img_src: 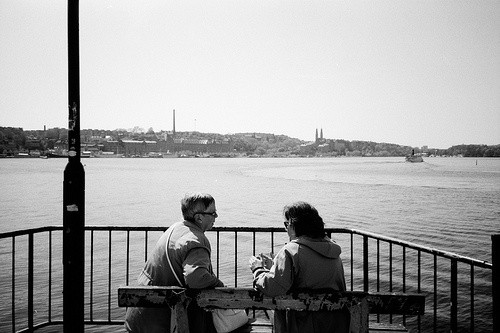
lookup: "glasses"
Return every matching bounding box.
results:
[284,221,290,228]
[193,210,216,218]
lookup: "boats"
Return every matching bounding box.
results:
[405,152,426,163]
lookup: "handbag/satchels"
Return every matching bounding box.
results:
[190,305,249,333]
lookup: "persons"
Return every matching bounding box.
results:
[247,199,351,333]
[123,192,251,333]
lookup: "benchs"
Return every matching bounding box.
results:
[117,286,427,333]
